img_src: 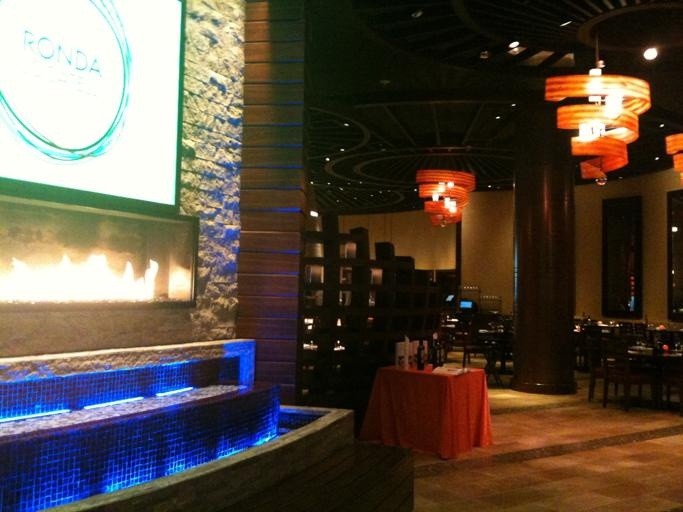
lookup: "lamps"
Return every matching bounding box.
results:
[413,150,476,230]
[542,32,653,187]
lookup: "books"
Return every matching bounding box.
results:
[431,363,470,376]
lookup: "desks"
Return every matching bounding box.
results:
[375,361,486,450]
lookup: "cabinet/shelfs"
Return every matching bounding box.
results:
[296,213,445,407]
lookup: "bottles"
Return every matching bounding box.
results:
[416,338,425,369]
[432,339,446,368]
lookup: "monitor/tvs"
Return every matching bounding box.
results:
[444,294,455,302]
[0,0,186,216]
[459,300,472,309]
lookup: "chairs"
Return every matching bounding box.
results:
[457,314,682,414]
